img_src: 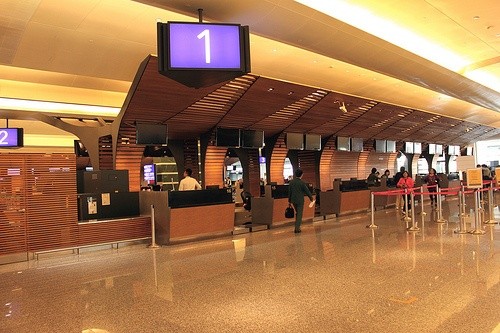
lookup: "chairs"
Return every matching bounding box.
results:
[241,191,255,212]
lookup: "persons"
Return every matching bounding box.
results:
[477,164,500,202]
[179,169,202,191]
[366,167,441,214]
[288,169,313,234]
[227,177,231,187]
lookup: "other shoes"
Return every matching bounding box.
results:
[402,211,405,215]
[435,204,437,206]
[408,209,412,213]
[431,201,433,204]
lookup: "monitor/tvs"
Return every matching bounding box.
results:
[74,140,89,157]
[336,136,351,151]
[157,21,251,88]
[135,124,168,146]
[241,130,264,148]
[386,141,396,153]
[0,128,23,148]
[143,164,156,185]
[305,133,321,151]
[350,138,363,152]
[405,142,422,154]
[215,127,241,148]
[373,140,386,153]
[428,144,474,156]
[285,133,304,150]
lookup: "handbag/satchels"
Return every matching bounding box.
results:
[285,205,294,218]
